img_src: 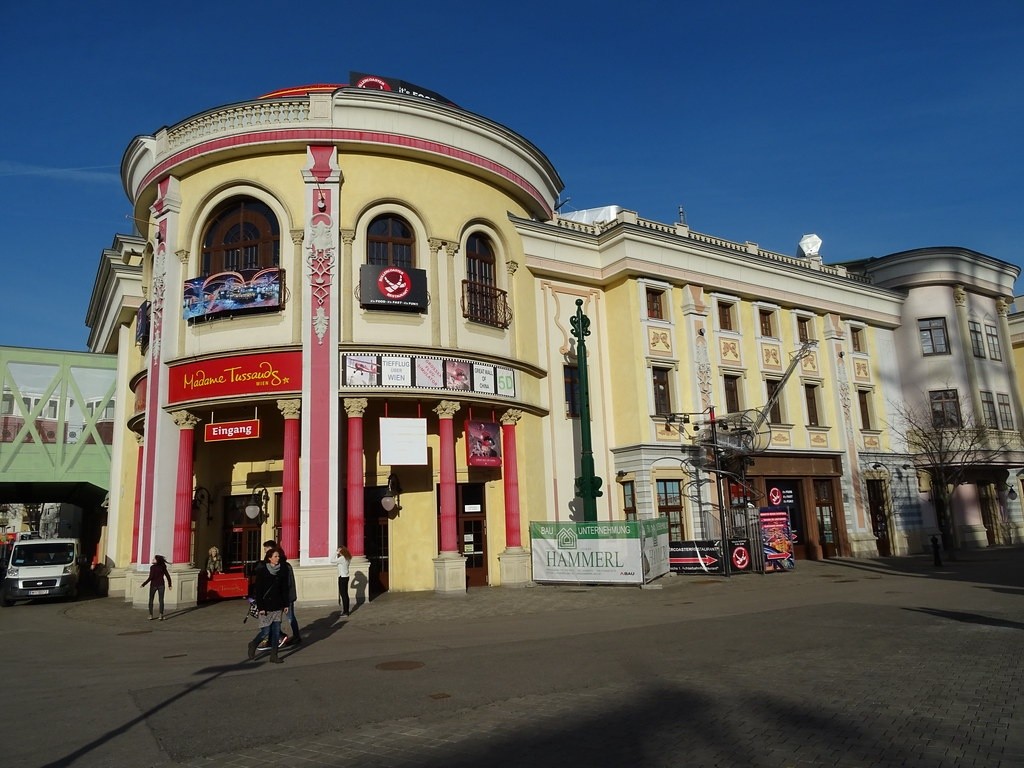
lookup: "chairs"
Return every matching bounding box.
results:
[52,548,69,559]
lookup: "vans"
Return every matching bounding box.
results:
[0,538,83,607]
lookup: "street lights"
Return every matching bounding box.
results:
[711,420,732,576]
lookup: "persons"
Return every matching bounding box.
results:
[140,555,172,620]
[205,547,225,579]
[930,534,944,567]
[248,548,290,663]
[256,540,302,650]
[330,545,352,617]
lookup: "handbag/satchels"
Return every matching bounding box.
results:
[250,603,259,618]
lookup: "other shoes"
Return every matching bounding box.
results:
[278,633,288,647]
[338,613,348,617]
[257,640,272,651]
[158,613,163,620]
[270,654,283,663]
[247,641,257,659]
[147,615,153,619]
[288,637,302,646]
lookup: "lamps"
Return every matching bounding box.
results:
[246,483,269,524]
[996,480,1017,500]
[901,464,910,470]
[872,464,881,470]
[381,473,402,517]
[192,486,214,526]
[618,470,627,477]
